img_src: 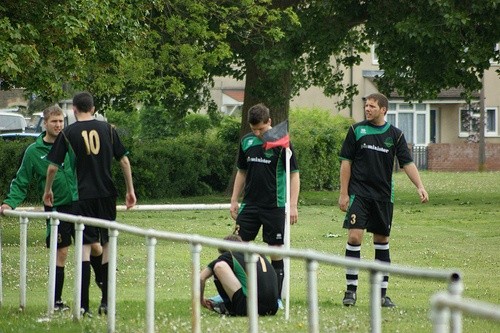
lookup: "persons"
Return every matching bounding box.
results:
[44,92,137,314]
[200,234,279,316]
[339,93,429,306]
[231,103,300,316]
[0,102,113,313]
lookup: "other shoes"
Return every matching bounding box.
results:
[208,296,224,305]
[53,301,70,312]
[343,290,357,305]
[381,296,395,306]
[213,302,230,316]
[278,299,284,309]
[81,308,93,319]
[99,304,107,315]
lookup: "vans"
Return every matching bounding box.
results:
[0,111,67,134]
